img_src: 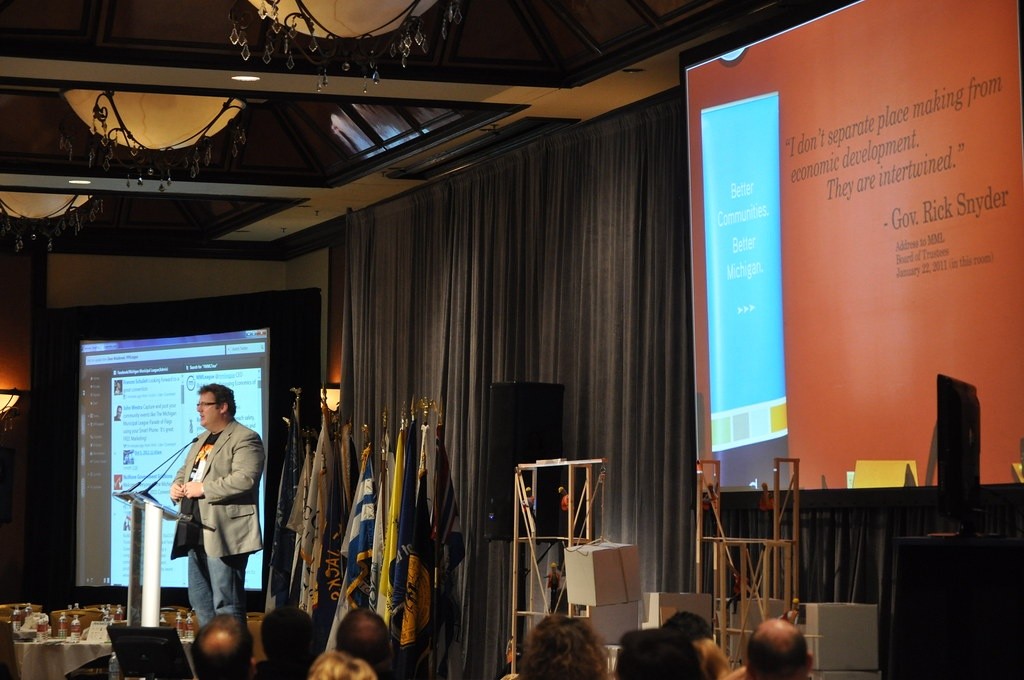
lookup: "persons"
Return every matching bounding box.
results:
[114,405,122,421]
[170,384,265,625]
[703,484,718,537]
[558,487,569,511]
[188,604,395,680]
[760,483,774,534]
[779,599,799,625]
[544,563,560,611]
[517,611,813,680]
[726,572,748,614]
[506,636,512,674]
[522,487,535,526]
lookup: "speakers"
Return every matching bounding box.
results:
[483,382,565,544]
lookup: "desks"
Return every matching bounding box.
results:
[888,536,1024,680]
[12,626,199,680]
[702,482,1024,623]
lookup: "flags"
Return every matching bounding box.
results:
[266,405,465,669]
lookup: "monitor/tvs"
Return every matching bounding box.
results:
[926,374,1003,537]
[107,624,194,680]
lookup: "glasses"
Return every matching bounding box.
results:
[197,402,222,407]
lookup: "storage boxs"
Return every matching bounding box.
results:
[564,542,883,680]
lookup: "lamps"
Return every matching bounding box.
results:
[0,190,105,251]
[57,87,248,191]
[228,0,466,94]
[0,387,25,431]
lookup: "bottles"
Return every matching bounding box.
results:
[109,652,120,680]
[25,603,32,618]
[185,613,194,640]
[58,613,68,641]
[13,606,21,632]
[68,605,72,610]
[116,605,123,614]
[73,603,80,610]
[71,615,81,643]
[106,604,111,611]
[101,605,105,611]
[102,611,110,622]
[160,615,166,622]
[176,612,183,639]
[37,614,48,642]
[246,613,249,621]
[110,615,114,622]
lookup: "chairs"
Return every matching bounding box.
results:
[0,603,268,680]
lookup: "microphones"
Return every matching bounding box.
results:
[121,437,199,505]
